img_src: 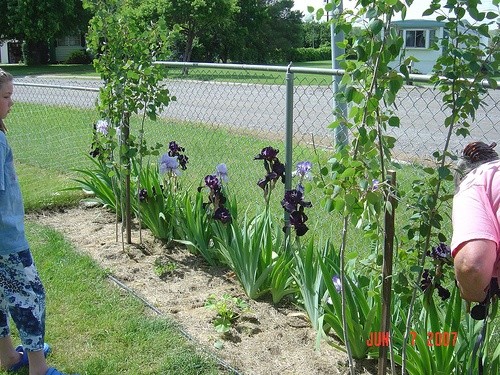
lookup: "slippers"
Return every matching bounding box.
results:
[6,343,51,372]
[46,365,79,375]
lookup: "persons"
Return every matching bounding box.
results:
[0,69,64,375]
[450,141,500,304]
